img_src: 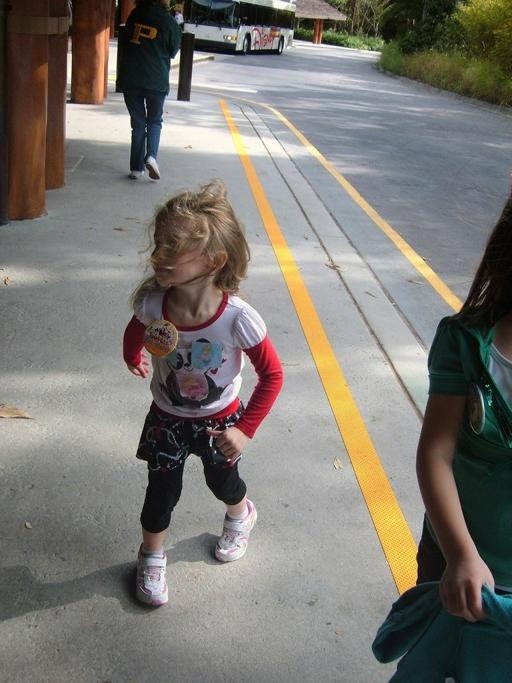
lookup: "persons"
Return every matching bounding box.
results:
[174,8,184,32]
[112,0,182,180]
[413,188,510,637]
[121,177,285,612]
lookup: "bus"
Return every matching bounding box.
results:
[185,0,297,56]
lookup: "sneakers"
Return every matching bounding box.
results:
[145,155,161,180]
[135,542,168,606]
[214,498,256,562]
[131,170,145,178]
